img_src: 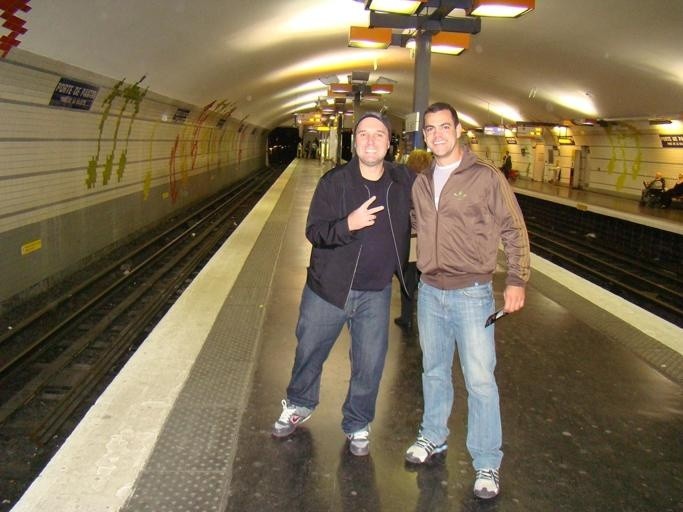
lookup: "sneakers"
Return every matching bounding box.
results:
[403,430,450,465]
[344,422,370,457]
[471,465,500,500]
[270,402,315,440]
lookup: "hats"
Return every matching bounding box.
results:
[353,111,393,144]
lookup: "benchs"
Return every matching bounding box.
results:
[641,190,683,210]
[331,157,348,166]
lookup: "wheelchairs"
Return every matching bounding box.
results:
[640,179,671,209]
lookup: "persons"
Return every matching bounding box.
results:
[309,142,318,157]
[304,140,310,158]
[404,102,532,499]
[395,149,431,327]
[271,111,418,459]
[297,140,303,158]
[501,150,512,178]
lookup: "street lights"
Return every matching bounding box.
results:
[348,0,535,153]
[322,81,393,159]
